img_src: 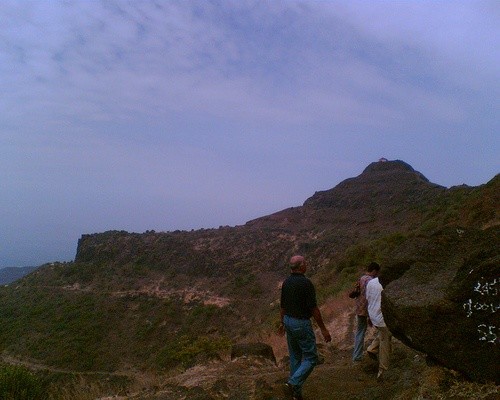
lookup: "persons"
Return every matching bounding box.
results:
[278,255,332,400]
[365,277,392,380]
[352,262,380,361]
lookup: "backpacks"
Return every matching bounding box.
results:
[348,276,368,299]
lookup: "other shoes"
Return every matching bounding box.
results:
[292,385,303,400]
[281,383,295,399]
